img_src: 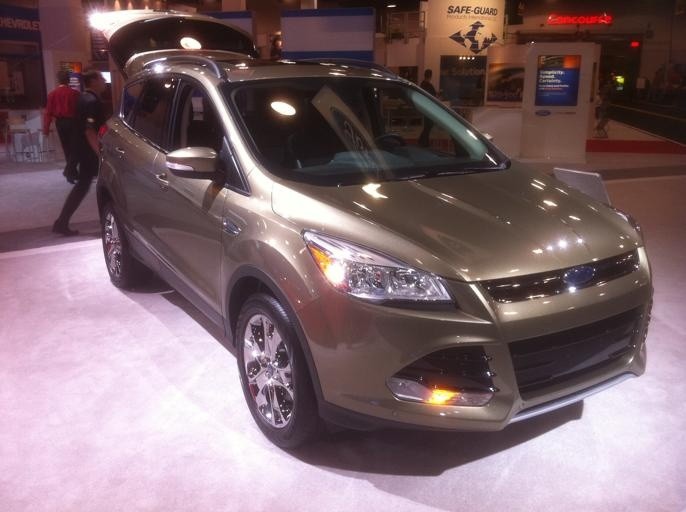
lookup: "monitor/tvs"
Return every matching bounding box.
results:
[440,55,486,100]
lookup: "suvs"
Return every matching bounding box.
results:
[88,4,657,454]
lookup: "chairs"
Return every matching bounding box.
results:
[0,110,57,164]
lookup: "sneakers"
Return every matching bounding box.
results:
[53,221,78,235]
[63,168,78,185]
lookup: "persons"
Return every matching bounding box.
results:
[51,69,107,239]
[415,69,438,149]
[39,71,82,185]
